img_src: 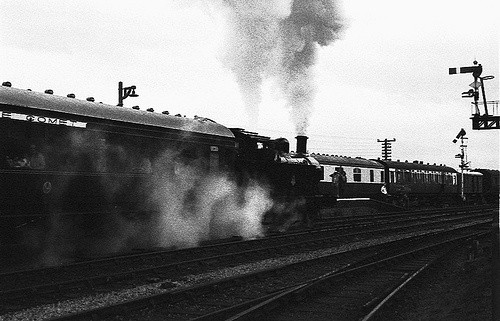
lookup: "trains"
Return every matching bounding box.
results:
[308,153,499,207]
[0,82,337,261]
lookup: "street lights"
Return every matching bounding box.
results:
[117,81,138,106]
